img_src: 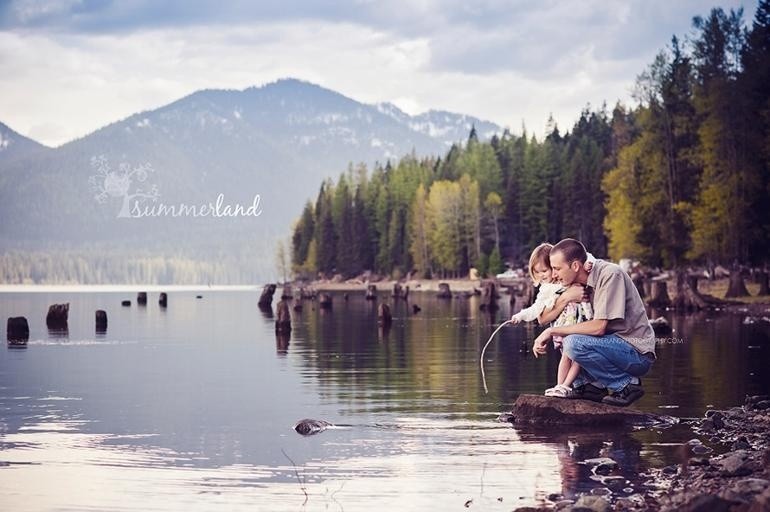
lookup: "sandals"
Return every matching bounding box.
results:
[545,383,573,398]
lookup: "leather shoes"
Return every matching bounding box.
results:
[568,383,644,406]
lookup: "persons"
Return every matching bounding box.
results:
[509,238,657,406]
